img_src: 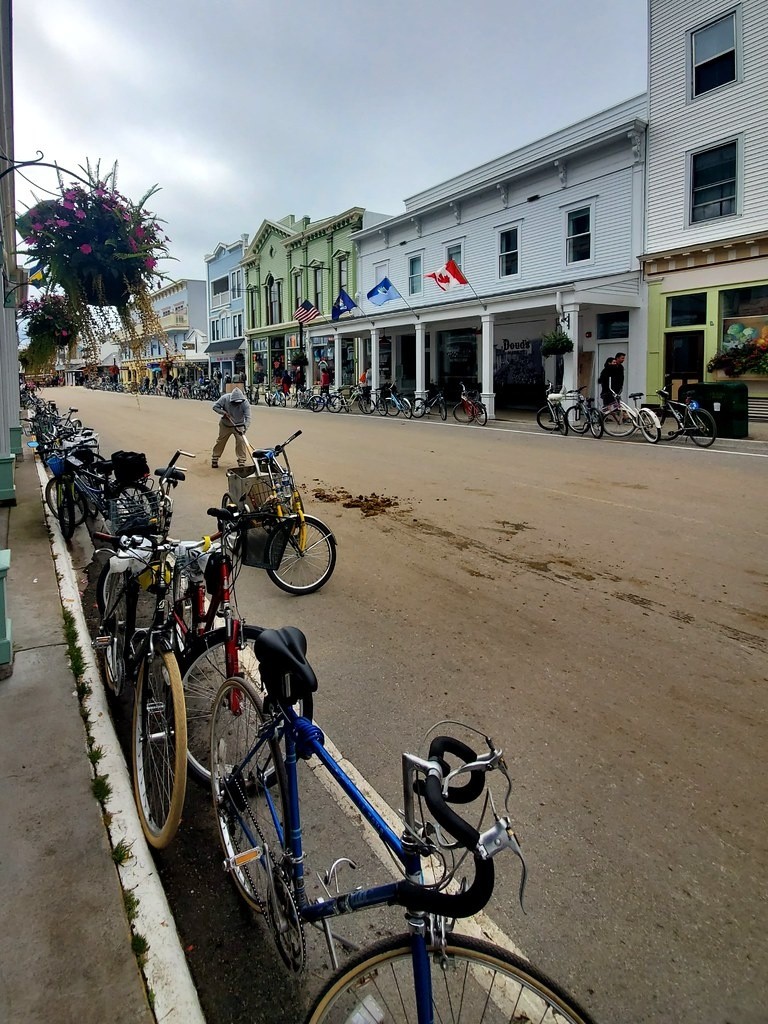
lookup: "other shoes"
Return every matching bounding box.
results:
[212,462,218,468]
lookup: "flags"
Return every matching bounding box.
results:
[424,259,467,292]
[30,261,48,289]
[367,276,401,305]
[331,288,358,321]
[292,299,320,323]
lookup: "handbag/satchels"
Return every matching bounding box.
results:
[111,450,150,483]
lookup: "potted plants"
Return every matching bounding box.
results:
[291,352,308,366]
[541,331,574,357]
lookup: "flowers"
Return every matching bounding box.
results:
[6,155,205,380]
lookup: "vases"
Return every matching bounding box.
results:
[20,358,28,366]
[54,329,71,347]
[82,276,129,308]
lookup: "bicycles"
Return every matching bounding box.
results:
[452,380,487,426]
[307,383,351,413]
[642,382,718,449]
[410,389,447,421]
[208,624,599,1024]
[217,429,337,595]
[564,385,605,439]
[19,374,323,853]
[324,382,368,413]
[536,380,569,436]
[357,387,388,417]
[383,378,412,419]
[600,377,662,444]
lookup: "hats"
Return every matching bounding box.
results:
[235,399,242,402]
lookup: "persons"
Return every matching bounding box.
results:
[19,374,64,392]
[86,366,247,394]
[359,368,371,405]
[212,387,251,469]
[254,359,330,403]
[600,352,632,425]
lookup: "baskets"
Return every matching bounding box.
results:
[22,423,36,436]
[109,489,161,534]
[351,387,363,396]
[47,456,69,483]
[248,476,286,510]
